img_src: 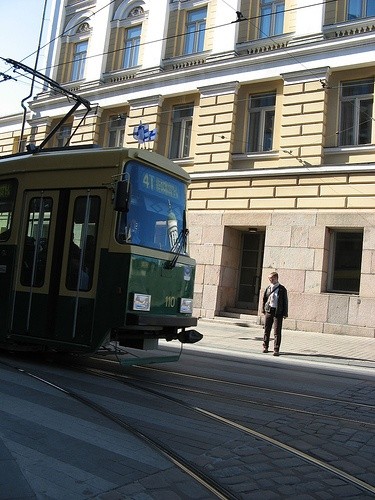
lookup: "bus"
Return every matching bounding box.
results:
[0,141,205,368]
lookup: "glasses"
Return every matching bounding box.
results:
[268,277,275,279]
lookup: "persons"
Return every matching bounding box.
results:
[261,271,288,357]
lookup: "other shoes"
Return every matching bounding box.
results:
[273,351,280,356]
[263,349,268,353]
